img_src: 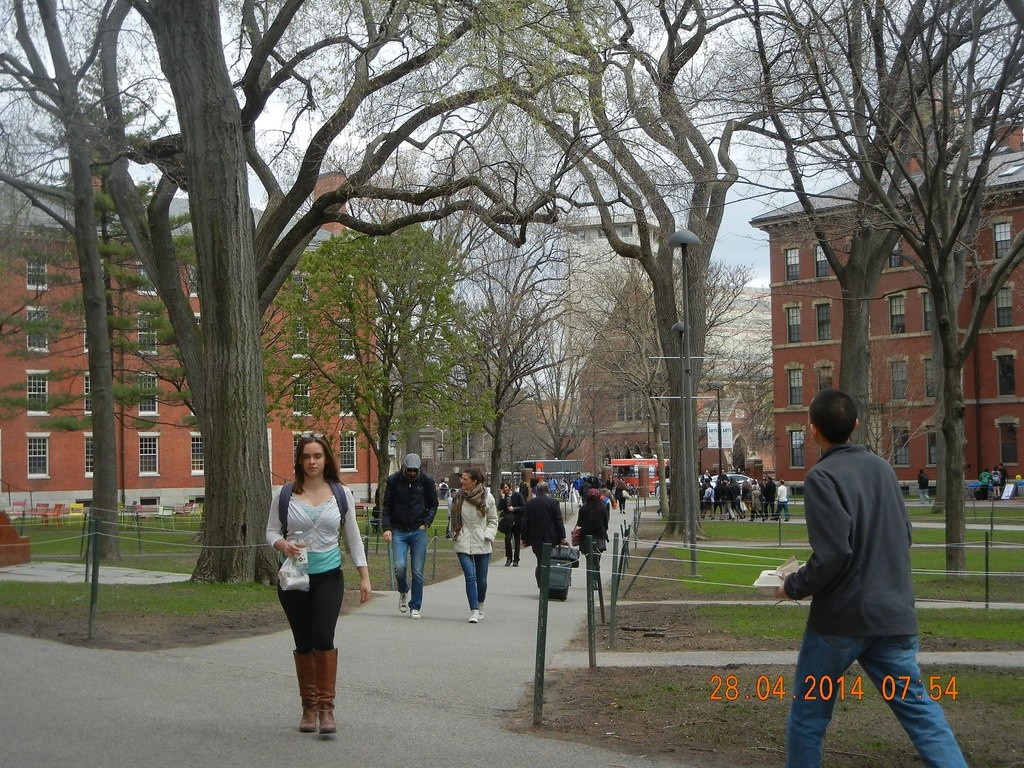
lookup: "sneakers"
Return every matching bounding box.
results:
[399,592,407,612]
[410,609,421,619]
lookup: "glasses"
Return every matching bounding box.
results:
[407,468,419,472]
[299,432,326,439]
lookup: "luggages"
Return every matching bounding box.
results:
[547,543,572,601]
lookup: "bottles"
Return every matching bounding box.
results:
[293,531,308,569]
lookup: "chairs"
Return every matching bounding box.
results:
[11,498,204,531]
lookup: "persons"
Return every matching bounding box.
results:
[656,486,661,516]
[530,473,539,495]
[918,469,931,504]
[577,489,609,590]
[497,482,529,566]
[446,489,456,540]
[265,436,371,734]
[979,463,1007,499]
[520,482,566,565]
[383,453,439,620]
[545,472,630,514]
[449,469,498,623]
[435,480,448,500]
[699,470,790,521]
[774,388,967,768]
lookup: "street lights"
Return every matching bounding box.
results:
[668,228,703,574]
[427,424,449,461]
[669,319,695,552]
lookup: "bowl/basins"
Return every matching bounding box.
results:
[753,570,784,595]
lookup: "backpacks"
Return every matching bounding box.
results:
[600,488,609,504]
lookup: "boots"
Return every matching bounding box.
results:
[313,648,338,732]
[293,649,318,732]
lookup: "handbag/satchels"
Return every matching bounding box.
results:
[552,541,580,568]
[613,500,618,510]
[740,500,747,512]
[497,515,515,533]
[622,490,630,498]
[702,497,711,506]
[571,524,582,546]
[278,556,310,592]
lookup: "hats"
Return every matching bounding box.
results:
[404,453,422,468]
[587,488,598,498]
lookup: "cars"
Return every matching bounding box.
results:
[699,473,759,490]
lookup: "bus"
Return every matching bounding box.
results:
[611,458,670,495]
[515,460,584,486]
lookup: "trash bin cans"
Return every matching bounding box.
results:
[927,486,937,497]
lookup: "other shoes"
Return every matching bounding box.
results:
[699,513,790,522]
[469,610,479,623]
[620,511,622,513]
[505,557,513,566]
[593,581,598,591]
[513,559,519,567]
[623,511,626,514]
[478,602,485,619]
